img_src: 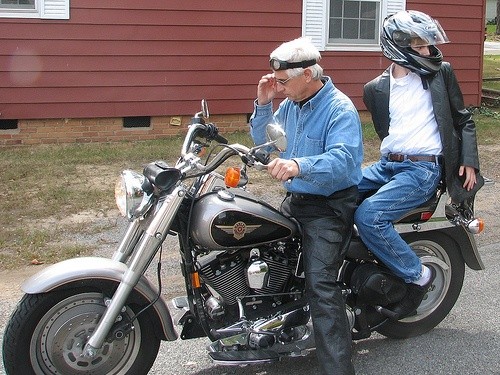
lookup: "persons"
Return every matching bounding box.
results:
[249,37,364,375]
[357,10,479,319]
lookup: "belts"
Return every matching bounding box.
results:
[389,153,440,162]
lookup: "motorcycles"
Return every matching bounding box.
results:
[2,99,497,375]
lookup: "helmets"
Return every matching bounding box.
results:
[380,10,451,78]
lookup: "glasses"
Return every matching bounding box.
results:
[268,59,315,71]
[275,77,290,86]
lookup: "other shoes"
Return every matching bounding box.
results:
[402,265,436,317]
